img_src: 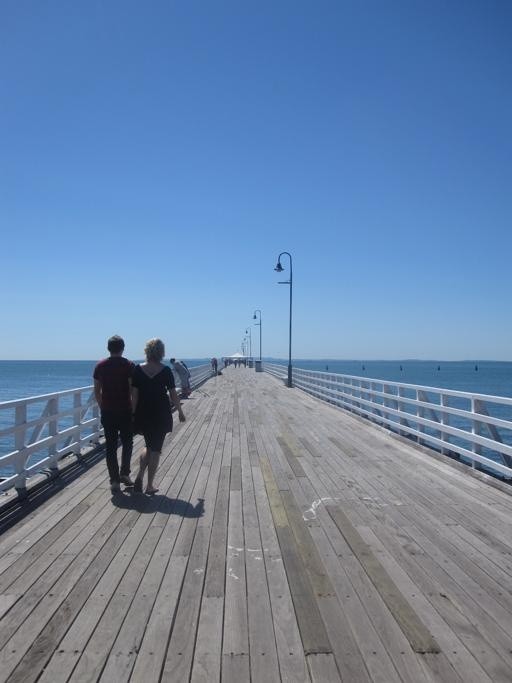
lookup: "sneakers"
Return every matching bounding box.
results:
[111,482,120,490]
[120,472,132,487]
[145,487,159,493]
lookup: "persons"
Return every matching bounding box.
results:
[94,335,135,491]
[132,337,185,494]
[170,357,247,398]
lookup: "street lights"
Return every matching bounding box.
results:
[241,251,293,388]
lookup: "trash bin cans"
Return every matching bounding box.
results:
[255,361,262,372]
[249,361,253,368]
